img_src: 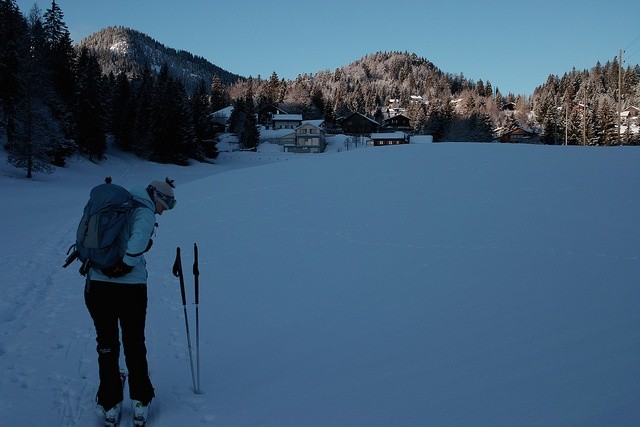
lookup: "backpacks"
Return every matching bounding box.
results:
[62,182,158,277]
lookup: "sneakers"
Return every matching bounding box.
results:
[131,400,150,427]
[104,403,121,426]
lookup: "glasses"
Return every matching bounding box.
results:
[147,185,177,209]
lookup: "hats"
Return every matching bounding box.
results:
[149,176,176,210]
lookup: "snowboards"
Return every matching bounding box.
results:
[105,369,149,427]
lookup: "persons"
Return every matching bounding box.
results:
[63,176,176,425]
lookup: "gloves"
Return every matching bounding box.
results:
[101,261,135,278]
